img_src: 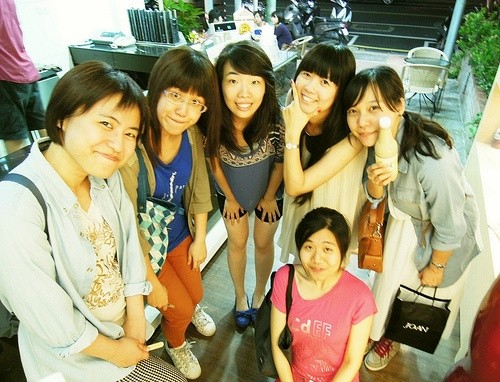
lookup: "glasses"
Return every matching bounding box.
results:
[163,89,208,114]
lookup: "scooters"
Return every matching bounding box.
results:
[287,0,353,46]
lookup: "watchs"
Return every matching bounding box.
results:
[285,143,300,150]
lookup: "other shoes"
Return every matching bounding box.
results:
[252,293,266,323]
[234,293,250,328]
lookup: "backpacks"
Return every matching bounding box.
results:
[0,171,47,338]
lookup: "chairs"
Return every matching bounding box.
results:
[401,47,449,112]
[286,35,313,58]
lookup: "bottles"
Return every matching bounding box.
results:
[374,116,399,182]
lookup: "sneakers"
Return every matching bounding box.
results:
[191,304,216,336]
[364,338,375,356]
[165,340,201,380]
[364,337,400,371]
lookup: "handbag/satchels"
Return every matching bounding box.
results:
[254,264,295,378]
[384,283,452,354]
[358,200,383,274]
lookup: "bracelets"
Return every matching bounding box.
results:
[363,179,387,204]
[430,258,445,269]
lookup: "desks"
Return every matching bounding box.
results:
[68,43,167,72]
[404,57,450,110]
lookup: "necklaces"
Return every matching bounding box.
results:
[305,129,311,137]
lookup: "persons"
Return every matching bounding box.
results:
[202,41,284,334]
[0,60,188,382]
[252,12,272,42]
[208,9,223,24]
[0,0,49,155]
[118,48,223,379]
[270,206,378,382]
[343,66,484,373]
[271,11,292,49]
[280,40,368,275]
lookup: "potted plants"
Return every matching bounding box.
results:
[162,0,209,45]
